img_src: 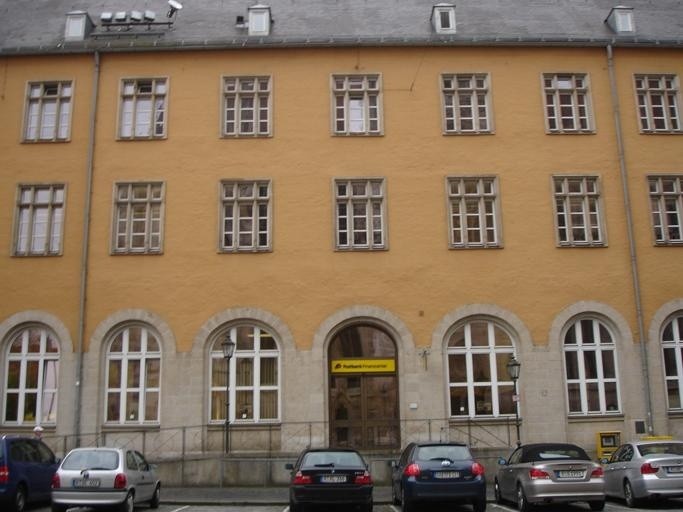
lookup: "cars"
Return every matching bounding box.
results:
[601,440,683,508]
[50,448,160,512]
[495,443,606,512]
[0,436,61,512]
[285,448,373,512]
[388,442,486,512]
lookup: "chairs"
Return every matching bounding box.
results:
[447,449,464,460]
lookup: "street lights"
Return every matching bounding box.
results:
[506,356,520,448]
[221,336,235,454]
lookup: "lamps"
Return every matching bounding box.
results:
[98,10,155,31]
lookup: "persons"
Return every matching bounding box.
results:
[31,426,44,440]
